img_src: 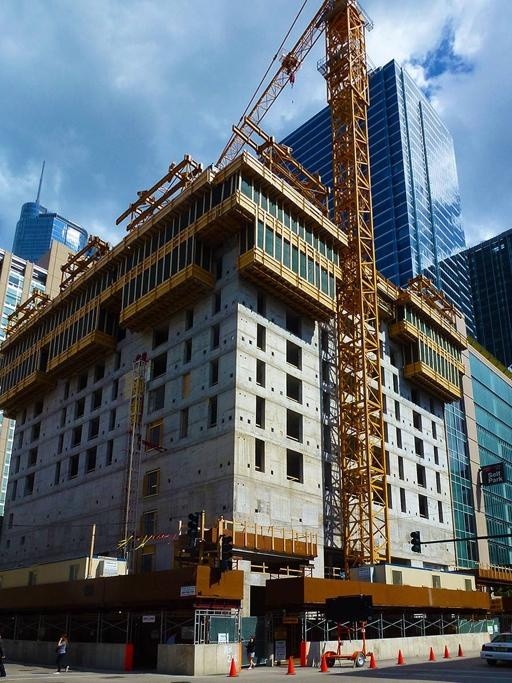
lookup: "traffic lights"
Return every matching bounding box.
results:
[410,531,421,553]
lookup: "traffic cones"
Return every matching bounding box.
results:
[318,655,330,672]
[285,656,296,675]
[367,644,466,669]
[226,658,239,677]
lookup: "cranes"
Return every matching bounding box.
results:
[214,0,392,581]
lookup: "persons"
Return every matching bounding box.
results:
[0,635,6,677]
[243,635,257,670]
[166,632,177,644]
[52,632,70,674]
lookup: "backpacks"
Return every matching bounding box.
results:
[65,641,72,656]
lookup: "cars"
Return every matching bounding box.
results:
[480,633,512,666]
